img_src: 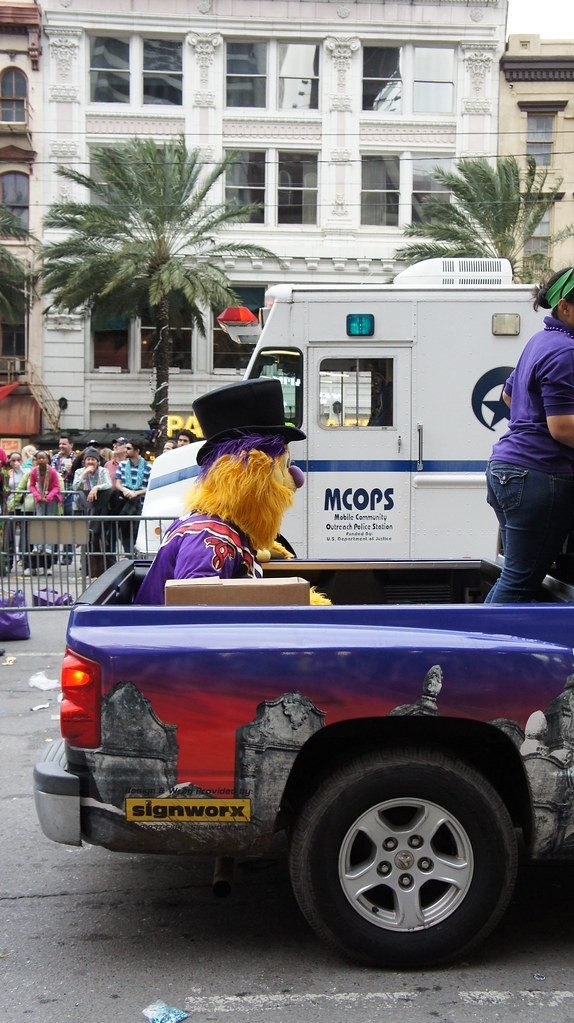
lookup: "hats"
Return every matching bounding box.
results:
[112,437,128,444]
[84,448,100,461]
[86,439,100,448]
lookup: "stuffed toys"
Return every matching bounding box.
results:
[132,379,334,606]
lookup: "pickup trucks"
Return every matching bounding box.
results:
[33,555,574,967]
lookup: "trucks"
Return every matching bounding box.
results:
[133,258,573,603]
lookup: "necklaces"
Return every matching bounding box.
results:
[544,327,574,339]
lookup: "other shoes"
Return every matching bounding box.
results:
[45,549,51,553]
[23,568,35,575]
[36,567,52,575]
[32,548,41,553]
[61,559,71,565]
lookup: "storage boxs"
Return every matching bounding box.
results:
[164,575,310,607]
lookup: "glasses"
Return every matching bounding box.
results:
[125,447,135,450]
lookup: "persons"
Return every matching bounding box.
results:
[0,432,152,577]
[483,267,574,603]
[155,429,195,458]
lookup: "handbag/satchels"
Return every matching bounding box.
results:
[0,589,30,640]
[33,587,72,606]
